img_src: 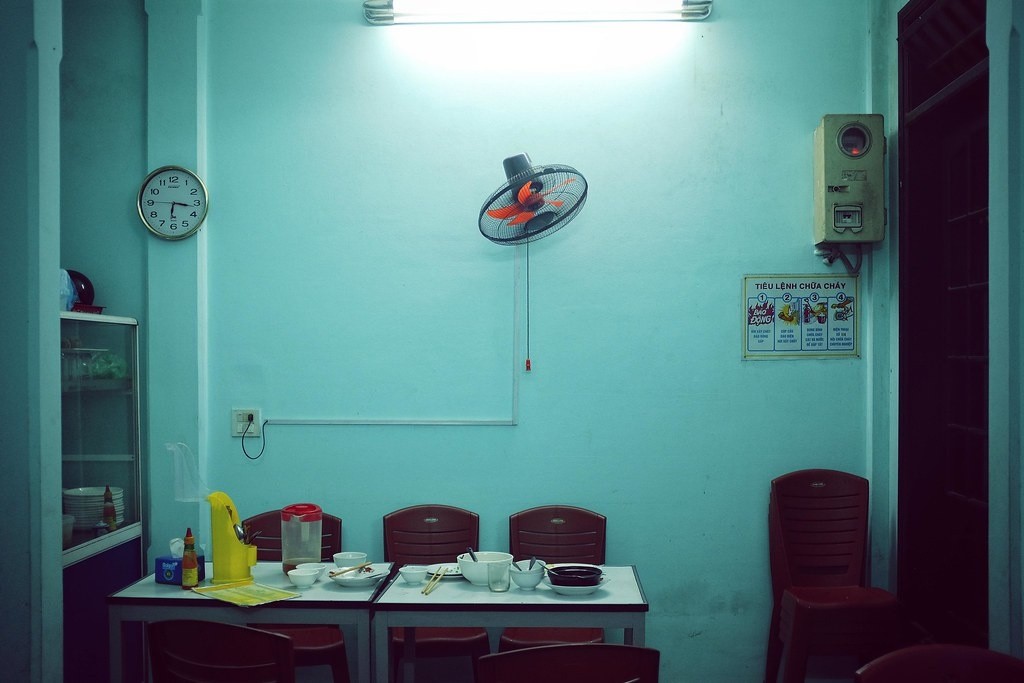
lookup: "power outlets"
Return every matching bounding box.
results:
[231,410,259,437]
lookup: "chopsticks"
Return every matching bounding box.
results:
[329,561,372,578]
[421,566,448,595]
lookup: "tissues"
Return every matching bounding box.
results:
[153,537,206,587]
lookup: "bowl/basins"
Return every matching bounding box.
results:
[288,562,326,588]
[398,567,428,586]
[511,559,548,591]
[457,552,514,587]
[333,552,367,567]
[548,566,602,586]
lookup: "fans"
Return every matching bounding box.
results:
[477,153,588,371]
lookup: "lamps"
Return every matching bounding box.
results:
[363,0,713,25]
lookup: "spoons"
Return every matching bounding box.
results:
[234,522,263,545]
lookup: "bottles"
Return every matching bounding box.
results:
[61,352,93,382]
[182,528,198,589]
[93,485,117,536]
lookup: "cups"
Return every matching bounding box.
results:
[487,563,510,592]
[62,516,76,546]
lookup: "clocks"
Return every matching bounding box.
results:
[137,165,209,241]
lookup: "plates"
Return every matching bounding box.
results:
[327,567,391,587]
[63,487,124,530]
[543,576,605,595]
[428,563,464,580]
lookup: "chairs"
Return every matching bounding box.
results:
[476,642,661,683]
[145,619,297,683]
[241,510,350,683]
[499,505,606,651]
[764,469,898,683]
[853,645,1024,683]
[382,505,490,683]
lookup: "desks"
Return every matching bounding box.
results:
[372,563,649,683]
[104,561,396,683]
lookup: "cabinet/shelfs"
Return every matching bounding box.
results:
[61,311,142,569]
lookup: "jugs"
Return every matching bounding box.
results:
[280,503,323,575]
